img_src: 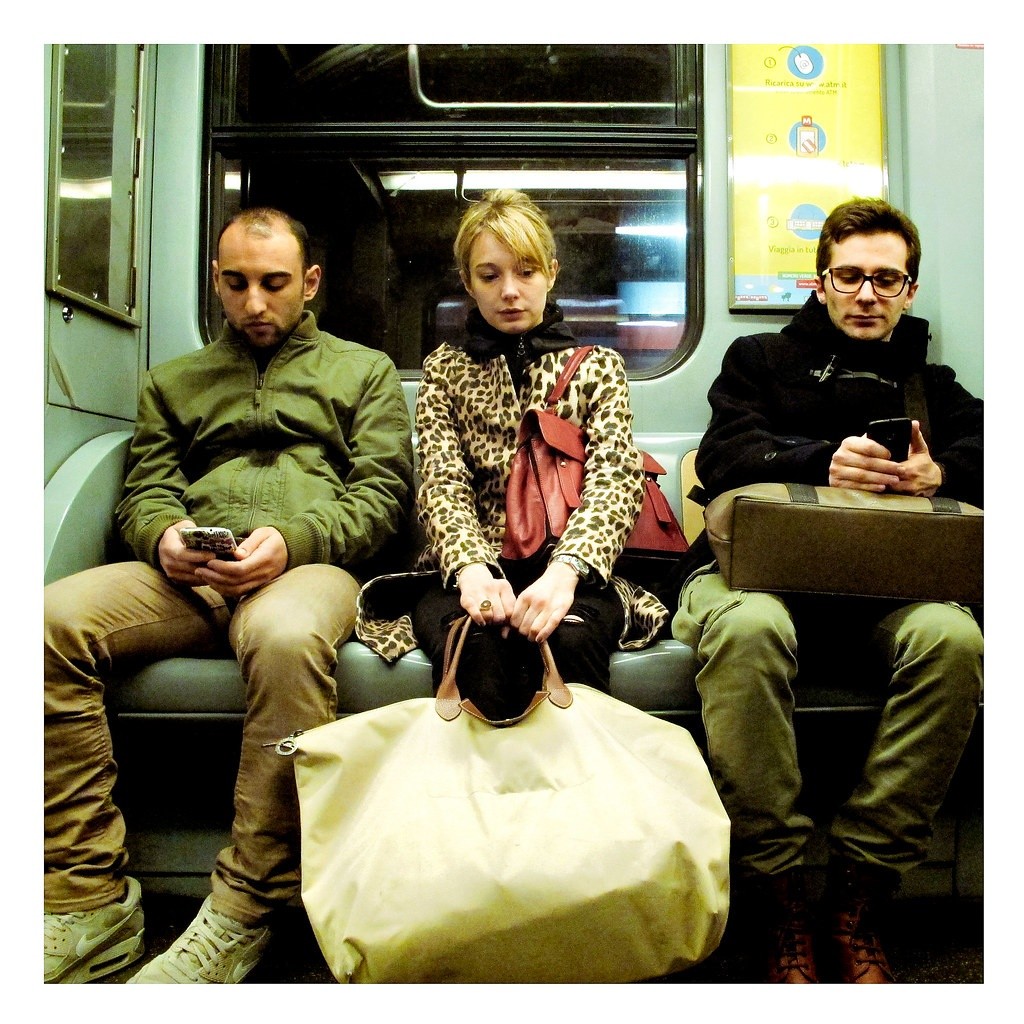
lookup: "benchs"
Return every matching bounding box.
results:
[44,431,881,723]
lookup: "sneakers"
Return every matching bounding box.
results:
[44,876,144,984]
[125,893,270,984]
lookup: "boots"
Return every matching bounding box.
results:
[748,859,899,984]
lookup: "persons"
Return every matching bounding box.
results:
[44,206,413,984]
[671,200,984,984]
[413,189,645,720]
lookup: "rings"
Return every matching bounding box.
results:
[480,600,494,611]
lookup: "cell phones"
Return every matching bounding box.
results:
[867,418,912,493]
[181,526,240,565]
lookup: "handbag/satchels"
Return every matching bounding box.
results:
[502,346,689,579]
[262,615,731,984]
[703,482,984,606]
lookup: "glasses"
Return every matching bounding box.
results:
[821,269,912,297]
